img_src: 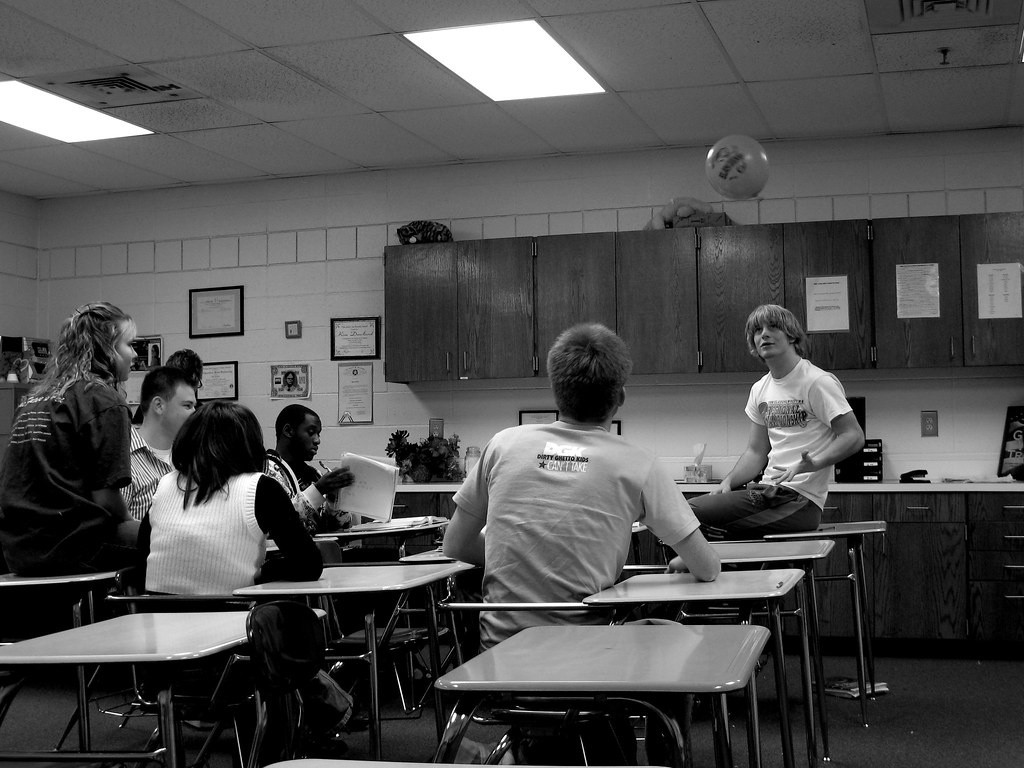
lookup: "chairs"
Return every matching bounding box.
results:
[104,559,263,768]
[244,601,332,758]
[306,536,448,718]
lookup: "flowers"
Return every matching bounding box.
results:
[387,431,460,472]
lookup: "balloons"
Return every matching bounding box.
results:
[705,135,770,199]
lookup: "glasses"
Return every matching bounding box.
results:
[196,377,203,388]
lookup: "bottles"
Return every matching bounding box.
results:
[463,446,481,477]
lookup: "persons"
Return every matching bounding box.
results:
[687,304,865,541]
[443,323,721,765]
[120,349,383,757]
[0,302,140,576]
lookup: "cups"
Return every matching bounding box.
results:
[350,512,361,532]
[685,467,708,483]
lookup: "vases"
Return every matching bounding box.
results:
[408,461,437,481]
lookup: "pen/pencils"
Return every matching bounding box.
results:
[319,460,332,473]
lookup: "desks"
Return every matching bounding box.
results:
[0,521,887,768]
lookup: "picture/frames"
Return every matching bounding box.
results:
[327,313,384,358]
[122,335,164,407]
[518,410,560,426]
[195,360,241,400]
[610,420,622,434]
[284,320,301,339]
[189,282,246,338]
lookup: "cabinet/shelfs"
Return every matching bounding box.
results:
[384,239,535,378]
[964,494,1024,660]
[869,211,1024,373]
[536,226,698,376]
[759,496,967,639]
[701,218,876,376]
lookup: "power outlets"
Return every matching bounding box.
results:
[430,418,444,438]
[920,409,938,438]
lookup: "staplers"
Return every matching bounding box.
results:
[898,469,931,483]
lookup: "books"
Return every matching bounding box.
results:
[811,674,891,698]
